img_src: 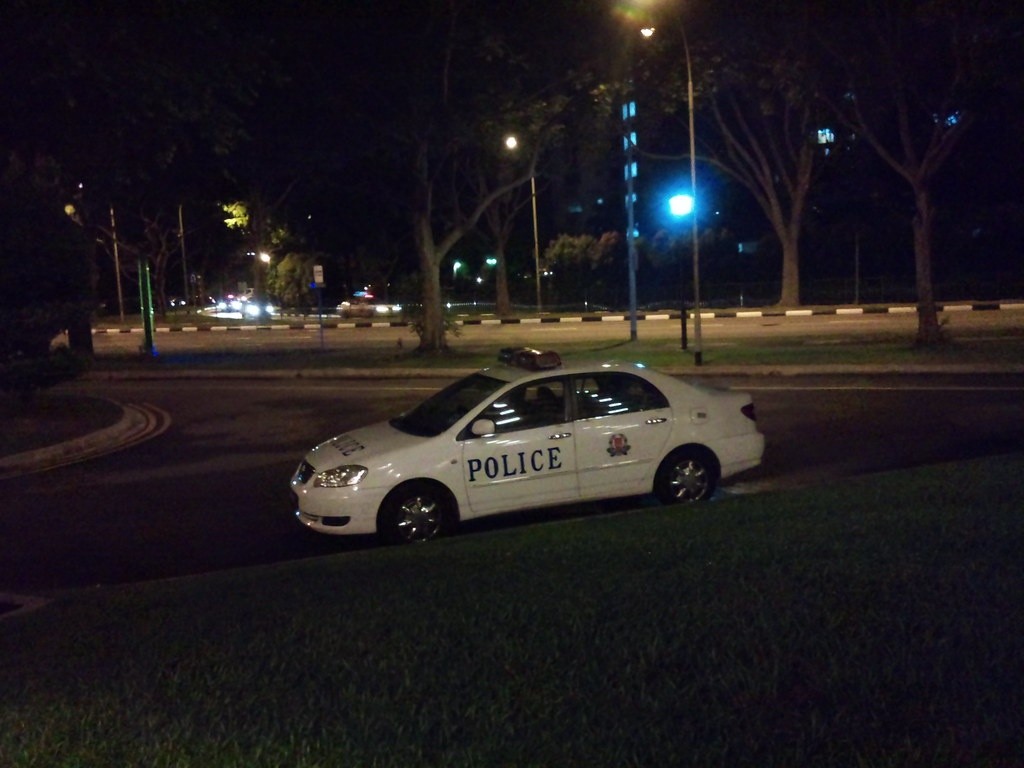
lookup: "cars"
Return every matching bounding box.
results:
[335,289,402,323]
[288,346,767,546]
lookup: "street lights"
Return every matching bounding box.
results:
[506,137,545,312]
[637,0,705,366]
